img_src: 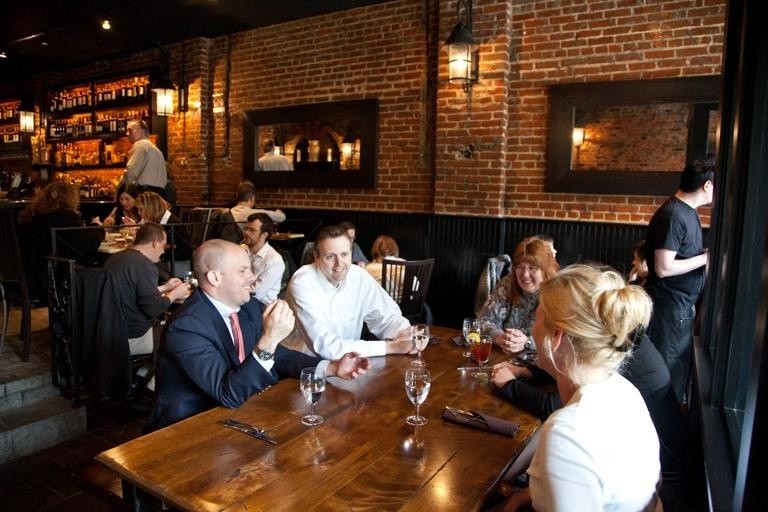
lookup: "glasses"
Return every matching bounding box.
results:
[513,264,541,273]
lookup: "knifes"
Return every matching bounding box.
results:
[217,419,278,445]
[455,364,493,370]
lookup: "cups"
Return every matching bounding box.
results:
[398,424,426,473]
[183,271,194,286]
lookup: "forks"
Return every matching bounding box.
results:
[226,418,270,437]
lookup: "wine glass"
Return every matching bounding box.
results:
[119,224,131,243]
[405,367,432,426]
[410,324,430,366]
[462,317,481,358]
[300,366,327,426]
[471,336,492,379]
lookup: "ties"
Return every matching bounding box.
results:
[229,313,245,362]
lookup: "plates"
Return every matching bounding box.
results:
[100,240,117,246]
[106,233,122,238]
[115,238,124,242]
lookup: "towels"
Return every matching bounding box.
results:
[450,333,470,347]
[428,333,445,346]
[442,405,520,438]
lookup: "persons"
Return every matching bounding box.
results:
[1,180,192,306]
[256,136,294,171]
[487,258,668,511]
[217,180,286,245]
[535,233,558,262]
[88,221,193,398]
[485,262,691,470]
[623,238,653,293]
[111,120,171,210]
[139,237,373,437]
[279,222,439,360]
[240,213,286,305]
[473,237,563,353]
[640,151,718,405]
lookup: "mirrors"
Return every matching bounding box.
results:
[243,97,379,190]
[544,74,722,196]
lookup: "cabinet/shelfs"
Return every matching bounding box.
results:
[0,97,42,197]
[43,66,167,201]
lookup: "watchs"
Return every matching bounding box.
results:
[524,335,534,352]
[254,344,281,363]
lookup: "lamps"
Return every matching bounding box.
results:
[439,0,479,92]
[148,48,190,117]
[341,125,358,160]
[17,80,46,133]
[571,126,586,167]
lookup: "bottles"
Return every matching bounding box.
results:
[55,141,79,167]
[47,76,149,138]
[0,103,28,161]
[55,172,113,198]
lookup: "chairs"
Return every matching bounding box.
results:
[0,207,434,424]
